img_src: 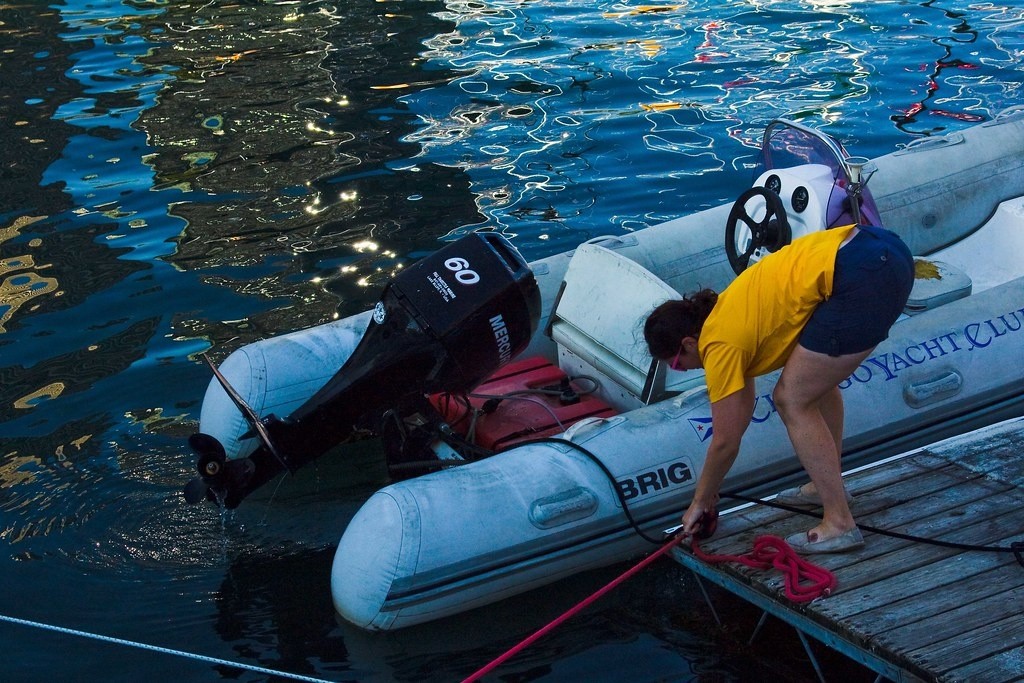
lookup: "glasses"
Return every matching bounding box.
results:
[670,344,687,372]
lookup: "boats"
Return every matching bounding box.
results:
[194,102,1024,635]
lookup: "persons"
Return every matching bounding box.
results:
[638,219,923,560]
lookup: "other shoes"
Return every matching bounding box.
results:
[783,523,864,553]
[776,484,855,509]
